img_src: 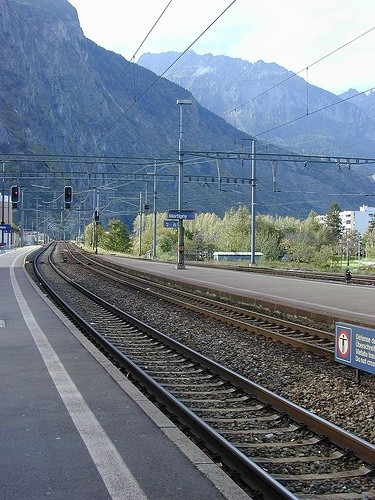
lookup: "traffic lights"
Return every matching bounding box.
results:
[11,187,19,202]
[65,187,72,202]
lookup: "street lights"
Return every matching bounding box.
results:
[175,98,193,269]
